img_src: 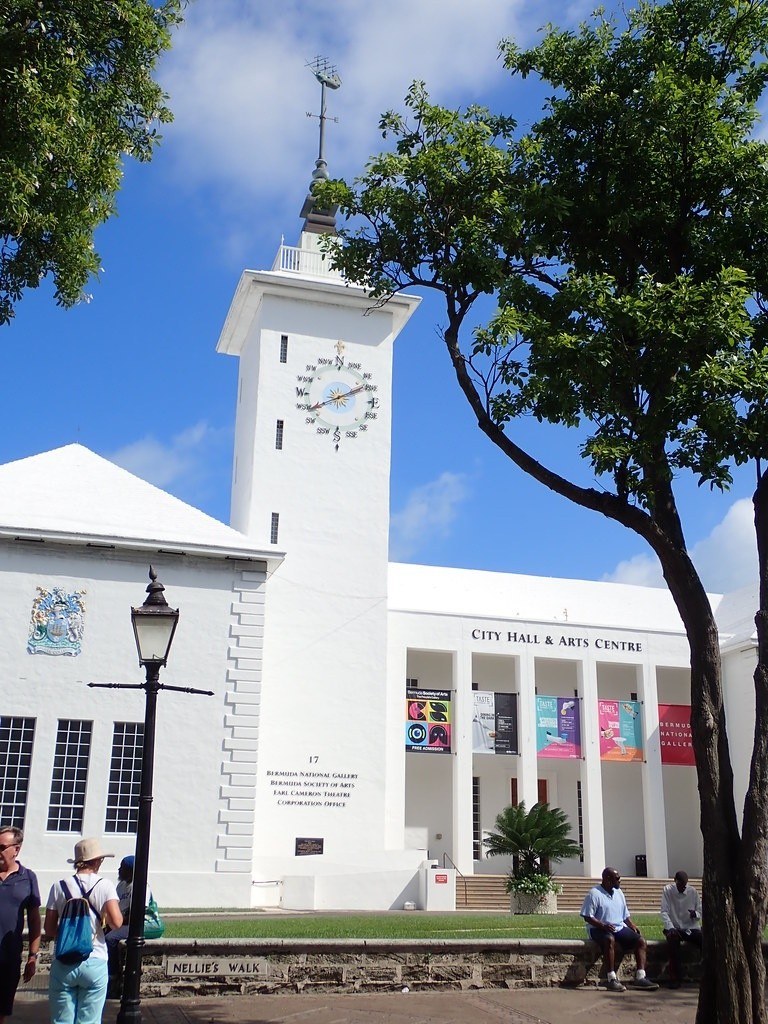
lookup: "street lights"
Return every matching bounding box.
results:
[85,565,215,1024]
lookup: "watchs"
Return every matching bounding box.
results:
[28,953,38,959]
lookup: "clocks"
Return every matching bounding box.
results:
[293,355,381,449]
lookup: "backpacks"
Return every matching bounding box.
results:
[54,875,103,965]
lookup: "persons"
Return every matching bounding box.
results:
[44,839,123,1024]
[102,855,151,999]
[580,866,659,992]
[661,871,702,989]
[0,826,42,1024]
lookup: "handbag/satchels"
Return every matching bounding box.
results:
[144,901,165,939]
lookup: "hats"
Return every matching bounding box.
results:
[67,838,115,863]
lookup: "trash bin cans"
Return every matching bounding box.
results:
[419,860,456,911]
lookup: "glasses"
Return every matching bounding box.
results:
[615,876,620,881]
[0,844,18,851]
[100,857,105,862]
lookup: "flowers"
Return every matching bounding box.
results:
[482,799,584,897]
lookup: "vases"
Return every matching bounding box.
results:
[509,890,558,915]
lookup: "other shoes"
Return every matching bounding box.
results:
[606,978,626,991]
[632,978,659,990]
[670,978,680,989]
